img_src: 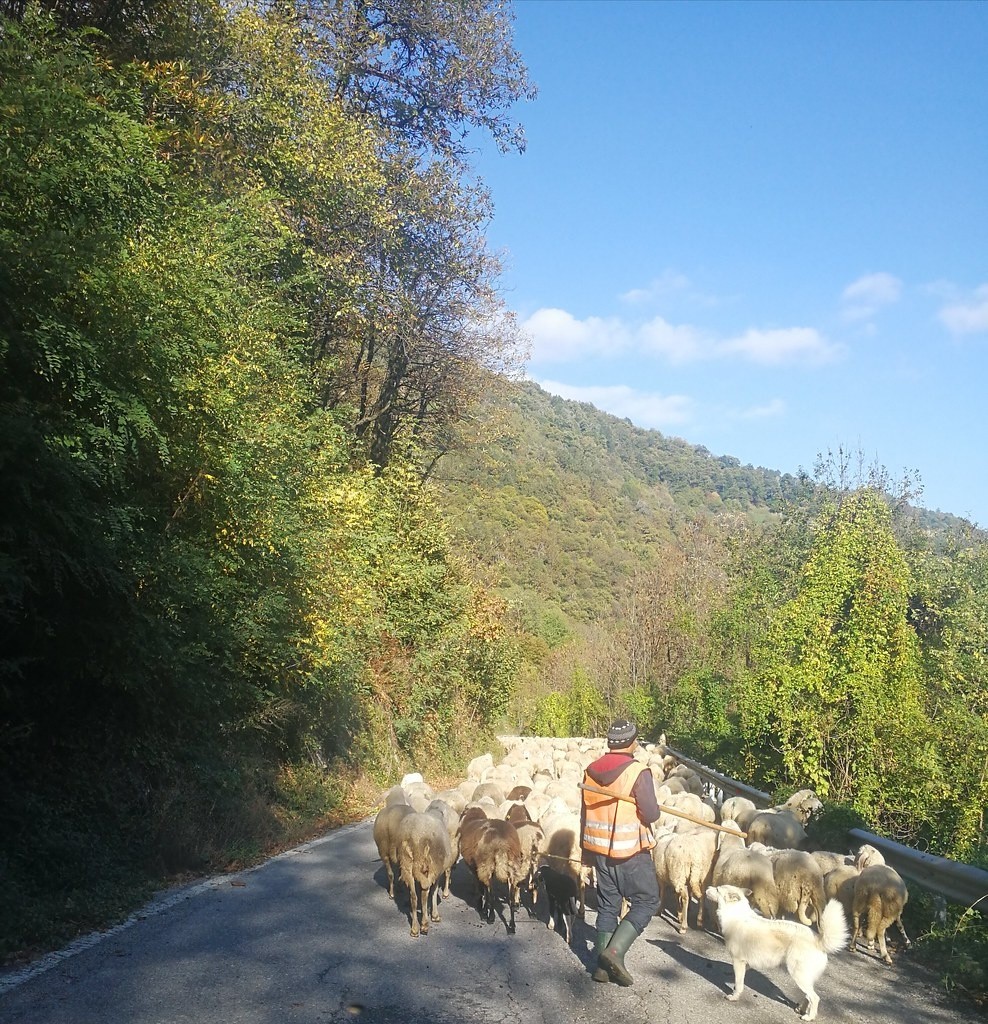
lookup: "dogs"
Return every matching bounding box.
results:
[706,884,851,1022]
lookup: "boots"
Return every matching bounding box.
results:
[591,932,615,983]
[600,919,634,987]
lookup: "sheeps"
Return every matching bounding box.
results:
[810,850,860,920]
[401,773,436,814]
[734,789,825,833]
[373,786,418,900]
[424,734,778,964]
[849,844,914,965]
[399,812,451,938]
[744,801,824,854]
[748,842,828,935]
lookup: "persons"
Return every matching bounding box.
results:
[580,720,661,985]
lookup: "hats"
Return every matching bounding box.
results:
[607,719,639,749]
